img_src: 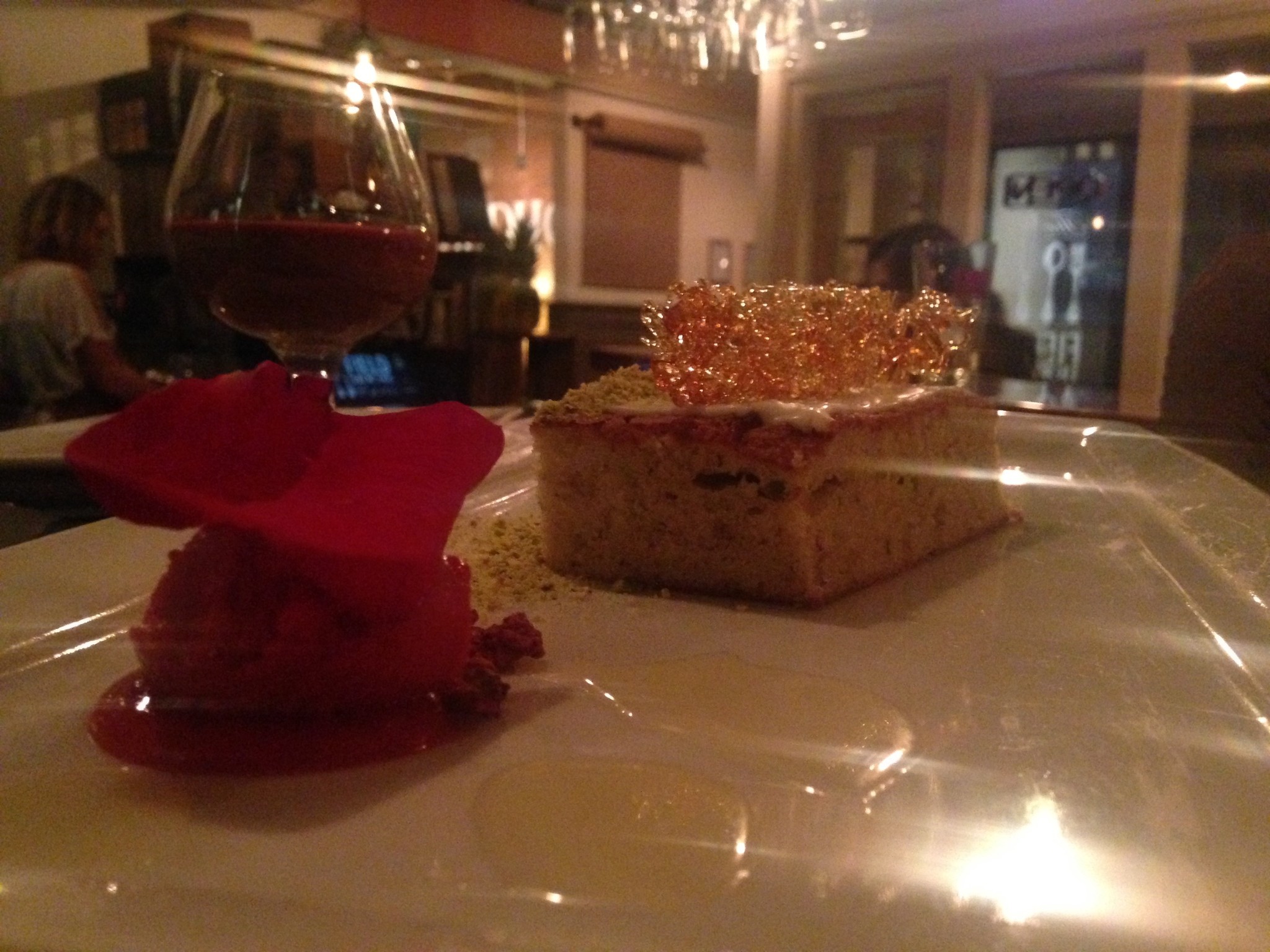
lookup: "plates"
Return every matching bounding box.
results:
[1,406,1270,951]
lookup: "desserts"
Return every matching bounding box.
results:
[60,360,507,718]
[529,280,1012,609]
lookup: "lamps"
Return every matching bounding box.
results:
[564,0,869,87]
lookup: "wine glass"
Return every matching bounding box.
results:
[164,67,440,411]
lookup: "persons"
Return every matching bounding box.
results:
[0,175,150,424]
